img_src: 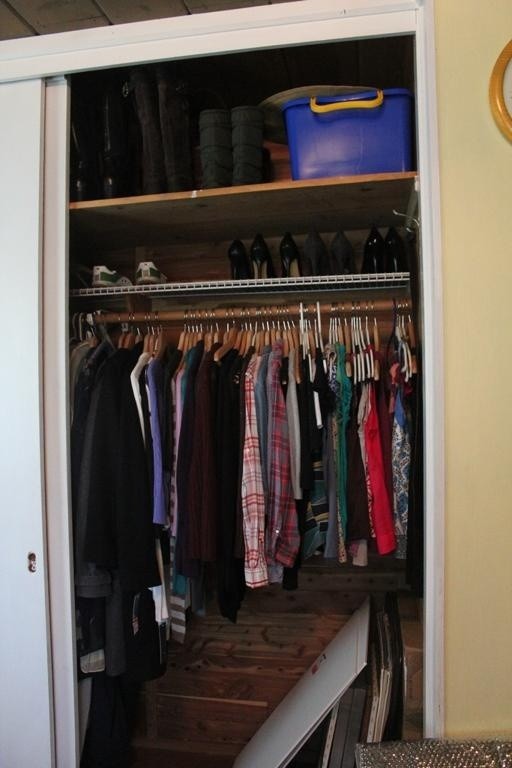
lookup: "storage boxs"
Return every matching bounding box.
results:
[284,88,408,181]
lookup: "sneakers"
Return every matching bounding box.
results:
[136,262,169,284]
[92,265,133,286]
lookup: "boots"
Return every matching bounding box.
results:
[70,80,266,202]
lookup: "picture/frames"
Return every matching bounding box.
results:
[490,40,512,142]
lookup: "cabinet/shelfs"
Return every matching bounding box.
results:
[0,1,443,767]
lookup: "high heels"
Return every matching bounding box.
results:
[227,228,405,279]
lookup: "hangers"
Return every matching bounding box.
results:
[68,299,417,385]
